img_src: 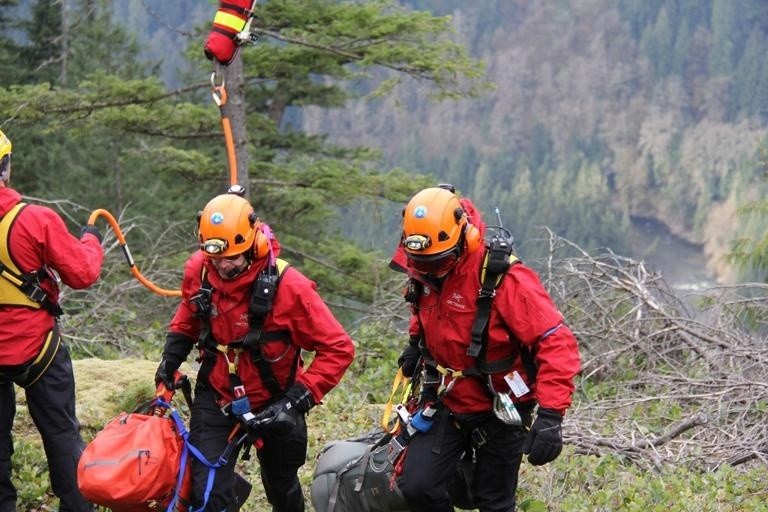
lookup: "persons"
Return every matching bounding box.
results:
[387,180,585,511]
[153,193,357,512]
[0,129,106,511]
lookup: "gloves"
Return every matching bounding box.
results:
[154,332,194,391]
[397,335,422,377]
[81,224,107,242]
[523,409,563,466]
[256,382,314,441]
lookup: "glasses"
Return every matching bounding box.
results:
[211,256,240,262]
[407,253,458,275]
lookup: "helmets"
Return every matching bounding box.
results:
[0,130,13,161]
[402,184,480,262]
[199,193,257,257]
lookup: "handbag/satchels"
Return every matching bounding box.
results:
[311,424,412,512]
[75,396,193,512]
